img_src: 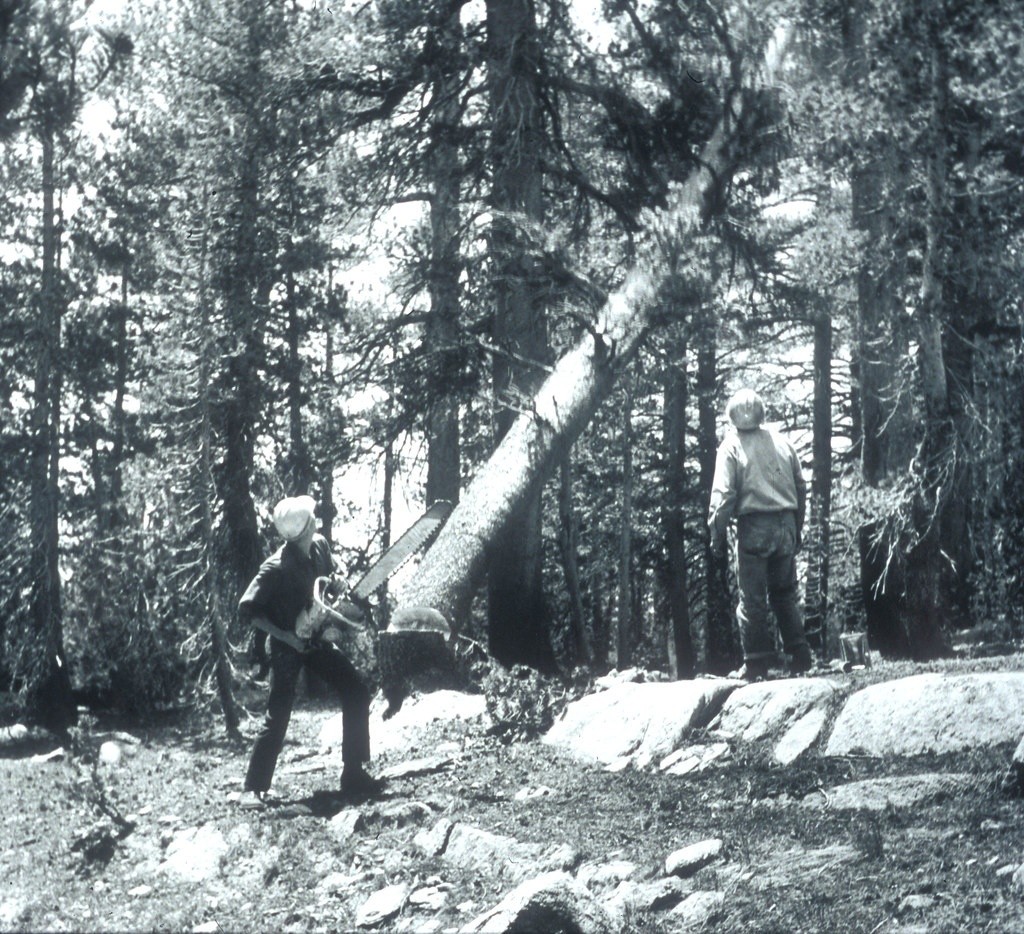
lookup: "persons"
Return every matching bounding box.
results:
[706,389,814,680]
[228,494,392,811]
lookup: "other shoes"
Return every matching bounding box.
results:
[238,791,265,810]
[342,768,386,788]
[728,647,811,680]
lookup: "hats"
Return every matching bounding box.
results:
[274,494,316,541]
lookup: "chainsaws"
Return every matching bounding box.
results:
[296,501,455,652]
